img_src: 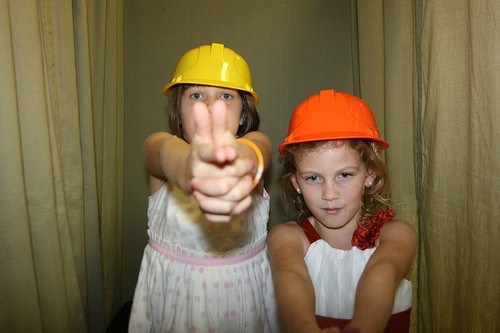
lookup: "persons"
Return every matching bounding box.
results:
[264,89,418,333]
[125,42,282,329]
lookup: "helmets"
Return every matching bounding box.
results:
[162,42,259,104]
[279,89,391,152]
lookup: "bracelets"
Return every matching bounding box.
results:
[236,138,265,191]
